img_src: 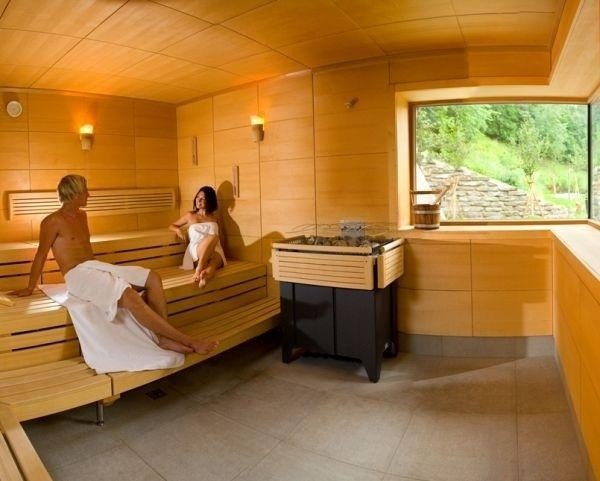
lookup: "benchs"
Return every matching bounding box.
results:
[0,226,189,294]
[0,258,267,371]
[0,292,283,432]
[0,401,57,481]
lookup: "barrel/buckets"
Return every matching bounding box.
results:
[409,188,442,229]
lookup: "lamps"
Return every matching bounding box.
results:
[79,124,94,151]
[250,115,264,141]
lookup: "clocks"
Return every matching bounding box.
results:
[6,101,23,118]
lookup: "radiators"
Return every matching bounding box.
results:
[7,187,176,221]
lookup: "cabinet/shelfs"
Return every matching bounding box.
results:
[278,281,400,384]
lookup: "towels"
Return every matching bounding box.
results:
[37,259,185,375]
[179,222,228,271]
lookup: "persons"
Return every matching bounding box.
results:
[169,186,228,289]
[5,175,220,356]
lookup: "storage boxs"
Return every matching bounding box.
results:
[270,235,404,291]
